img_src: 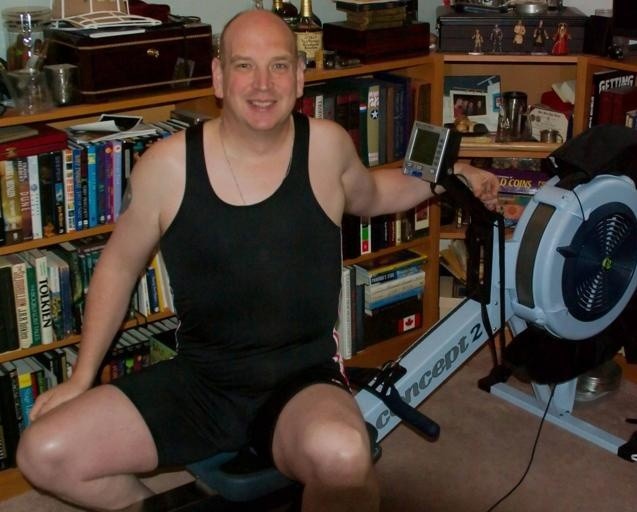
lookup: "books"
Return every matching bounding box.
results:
[1,109,213,248]
[1,234,177,355]
[337,248,427,360]
[291,76,431,169]
[341,210,415,261]
[1,316,180,470]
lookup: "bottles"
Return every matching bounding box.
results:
[269,0,324,68]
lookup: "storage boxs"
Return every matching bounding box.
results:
[436,4,591,55]
[322,19,432,64]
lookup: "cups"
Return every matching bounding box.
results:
[538,129,565,145]
[500,91,529,140]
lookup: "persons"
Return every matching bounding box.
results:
[15,6,500,511]
[454,97,485,116]
[470,19,573,56]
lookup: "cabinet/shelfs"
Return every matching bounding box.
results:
[0,51,637,506]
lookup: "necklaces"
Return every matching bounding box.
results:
[220,136,291,205]
